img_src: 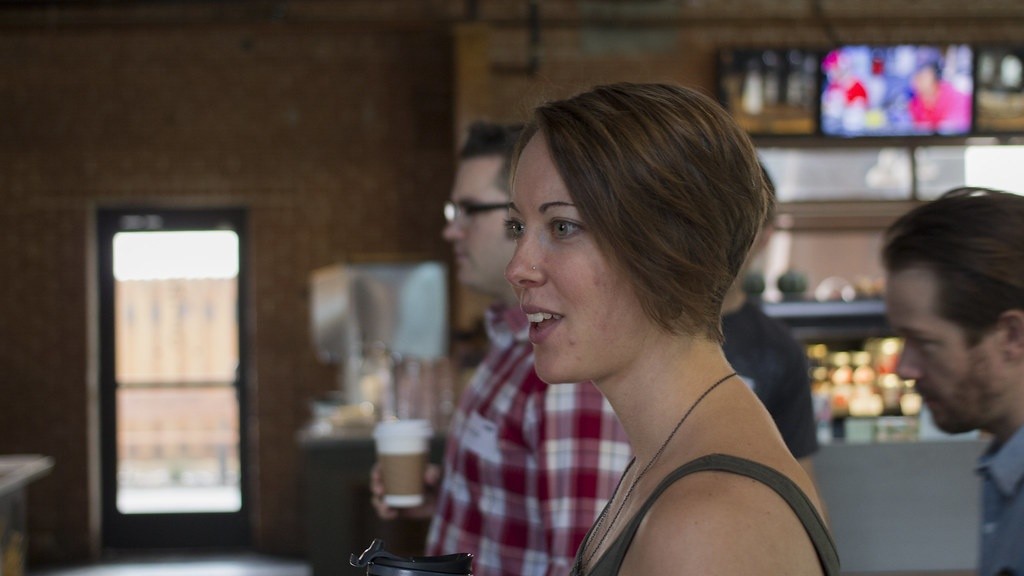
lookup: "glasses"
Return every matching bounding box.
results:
[438,198,515,226]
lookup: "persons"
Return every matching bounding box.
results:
[882,190,1024,576]
[904,59,972,137]
[424,82,840,574]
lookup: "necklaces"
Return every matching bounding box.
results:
[578,372,740,570]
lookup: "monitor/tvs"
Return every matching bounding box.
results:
[817,42,978,139]
